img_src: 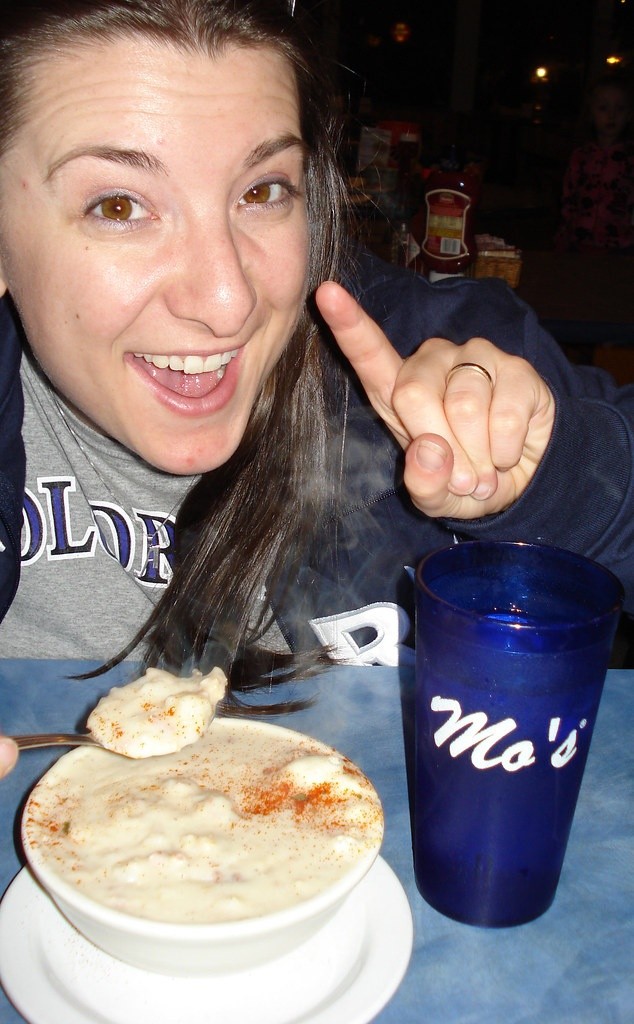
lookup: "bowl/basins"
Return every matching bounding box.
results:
[21,716,385,978]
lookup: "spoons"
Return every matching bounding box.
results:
[5,705,225,762]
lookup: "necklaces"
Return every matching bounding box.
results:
[48,390,198,577]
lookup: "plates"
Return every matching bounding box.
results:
[0,854,413,1024]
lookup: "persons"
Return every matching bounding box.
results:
[0,0,634,779]
[553,79,634,262]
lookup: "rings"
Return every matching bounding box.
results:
[446,362,495,390]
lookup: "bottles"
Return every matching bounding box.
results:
[397,133,419,184]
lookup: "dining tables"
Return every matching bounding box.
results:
[0,661,634,1024]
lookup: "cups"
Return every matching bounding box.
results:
[414,540,625,928]
